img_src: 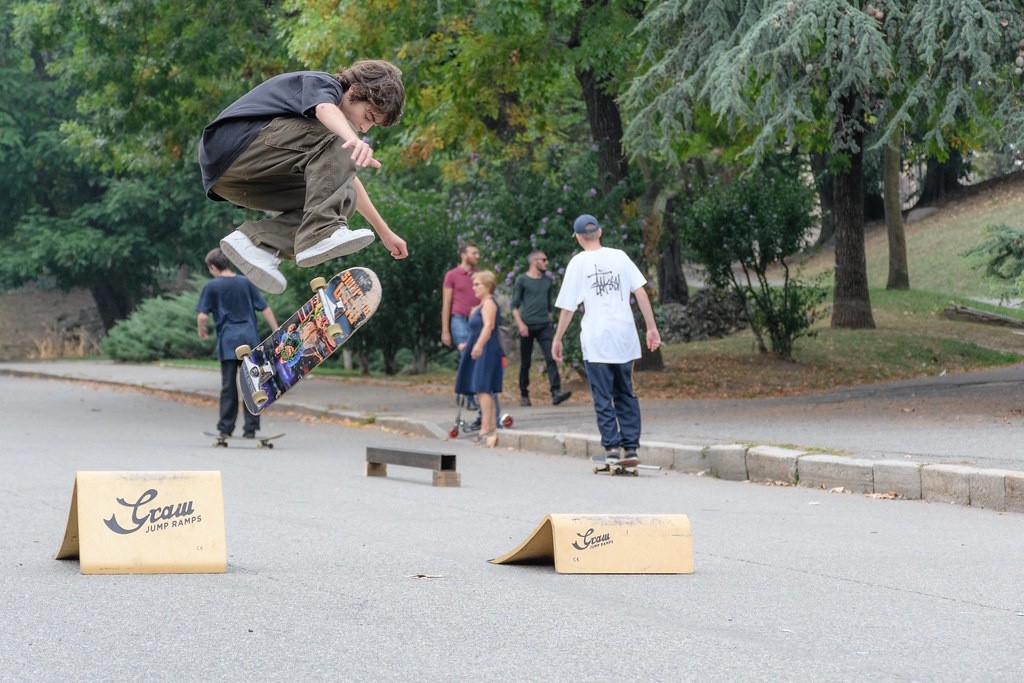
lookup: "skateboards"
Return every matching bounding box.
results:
[234,267,382,416]
[202,430,287,449]
[590,455,641,477]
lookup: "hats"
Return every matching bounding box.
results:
[571,214,599,237]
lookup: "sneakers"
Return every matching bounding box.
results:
[220,230,286,294]
[294,226,375,267]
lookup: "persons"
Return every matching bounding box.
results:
[455,269,504,447]
[440,243,502,431]
[552,214,661,462]
[199,61,409,295]
[513,252,572,407]
[198,247,277,439]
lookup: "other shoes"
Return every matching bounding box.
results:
[242,429,254,437]
[552,390,571,404]
[607,449,620,461]
[521,398,531,405]
[220,431,230,437]
[623,450,637,459]
[470,418,481,429]
